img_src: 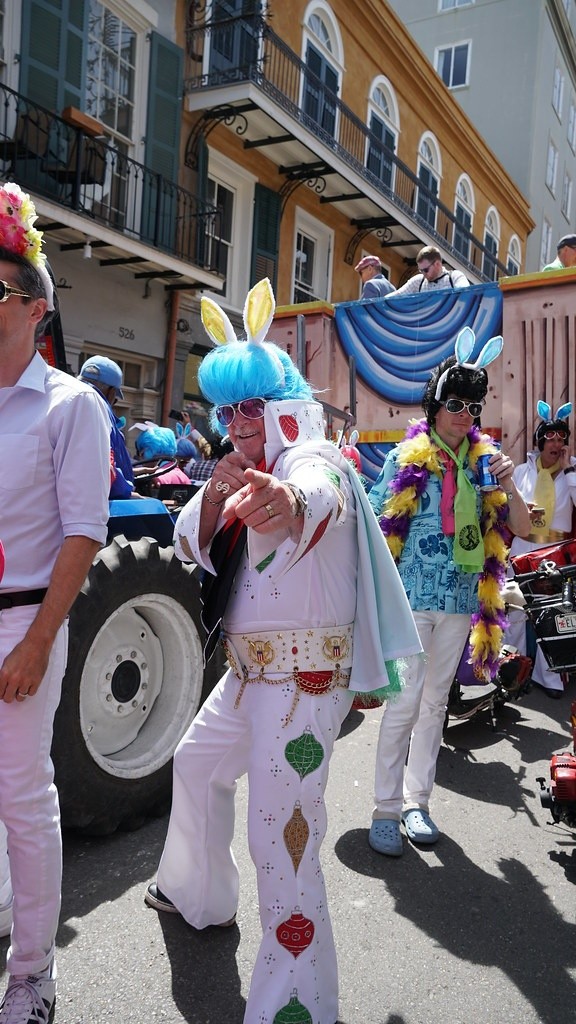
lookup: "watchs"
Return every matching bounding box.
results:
[287,483,309,519]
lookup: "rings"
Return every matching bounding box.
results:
[216,481,230,494]
[17,691,28,697]
[265,504,275,518]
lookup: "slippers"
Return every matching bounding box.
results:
[368,807,403,855]
[400,800,439,843]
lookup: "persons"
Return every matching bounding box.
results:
[542,233,576,271]
[354,255,397,301]
[0,181,114,1024]
[342,447,368,493]
[368,326,533,857]
[499,401,576,700]
[75,353,159,500]
[385,245,470,297]
[130,410,234,524]
[141,276,424,1024]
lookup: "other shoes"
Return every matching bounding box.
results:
[146,881,236,926]
[544,687,561,699]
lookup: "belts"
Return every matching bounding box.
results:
[0,587,48,612]
[219,624,357,681]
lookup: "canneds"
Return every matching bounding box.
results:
[477,454,500,491]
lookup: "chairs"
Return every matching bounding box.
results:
[0,108,56,180]
[44,137,107,206]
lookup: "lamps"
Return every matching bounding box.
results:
[83,240,92,259]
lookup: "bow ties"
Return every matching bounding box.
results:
[429,274,446,284]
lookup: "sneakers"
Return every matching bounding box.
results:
[0,895,15,938]
[0,955,58,1024]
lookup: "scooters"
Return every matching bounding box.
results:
[536,706,576,833]
[442,639,535,732]
[513,561,576,697]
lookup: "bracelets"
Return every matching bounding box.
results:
[203,492,222,507]
[501,482,515,502]
[564,466,574,475]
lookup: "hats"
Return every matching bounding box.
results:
[80,354,124,401]
[556,234,576,248]
[355,255,381,271]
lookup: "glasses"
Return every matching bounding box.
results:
[418,259,436,273]
[539,430,568,441]
[215,397,280,428]
[438,397,482,418]
[0,279,31,303]
